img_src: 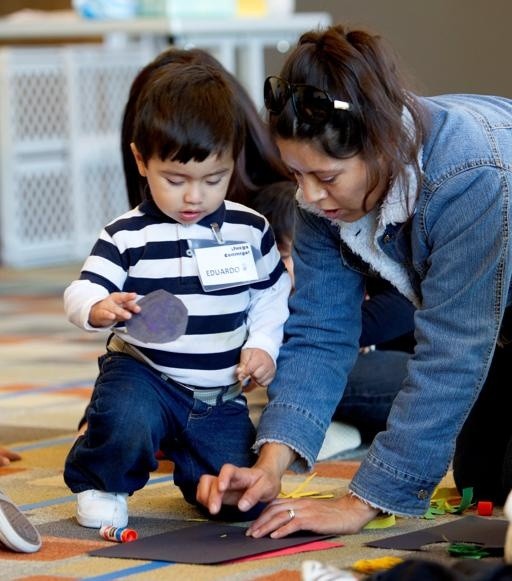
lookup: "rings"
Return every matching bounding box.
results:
[288,508,294,518]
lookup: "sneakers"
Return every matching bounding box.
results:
[76,489,128,530]
[0,495,41,554]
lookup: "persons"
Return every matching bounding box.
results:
[63,65,291,531]
[250,180,374,357]
[0,450,42,553]
[195,25,512,540]
[121,47,416,462]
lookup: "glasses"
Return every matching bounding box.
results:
[264,76,360,126]
